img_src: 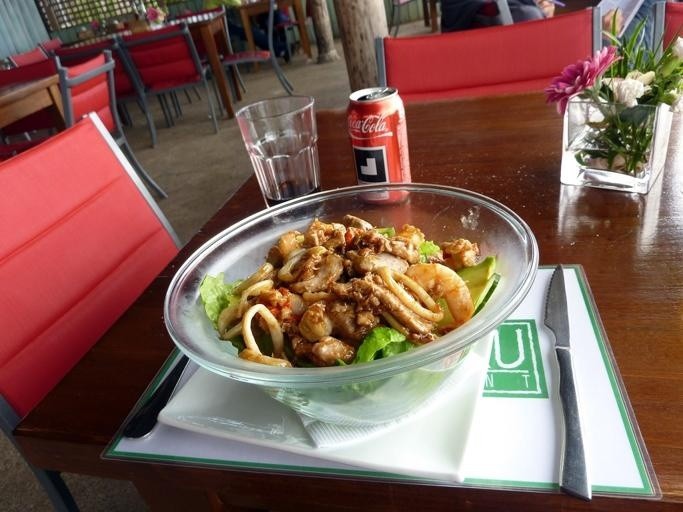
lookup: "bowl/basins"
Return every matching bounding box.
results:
[164,182,539,428]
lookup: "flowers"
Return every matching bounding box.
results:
[543,7,683,177]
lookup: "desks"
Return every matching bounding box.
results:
[10,105,681,512]
[4,65,66,147]
[67,10,241,119]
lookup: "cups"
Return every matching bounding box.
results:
[233,95,322,208]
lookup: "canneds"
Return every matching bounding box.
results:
[346,86,412,205]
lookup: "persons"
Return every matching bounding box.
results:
[441,0,555,32]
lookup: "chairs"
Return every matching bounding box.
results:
[37,36,63,56]
[1,48,62,128]
[9,47,48,66]
[57,50,168,202]
[114,23,225,148]
[1,109,200,500]
[370,6,602,102]
[48,40,175,148]
[219,3,295,97]
